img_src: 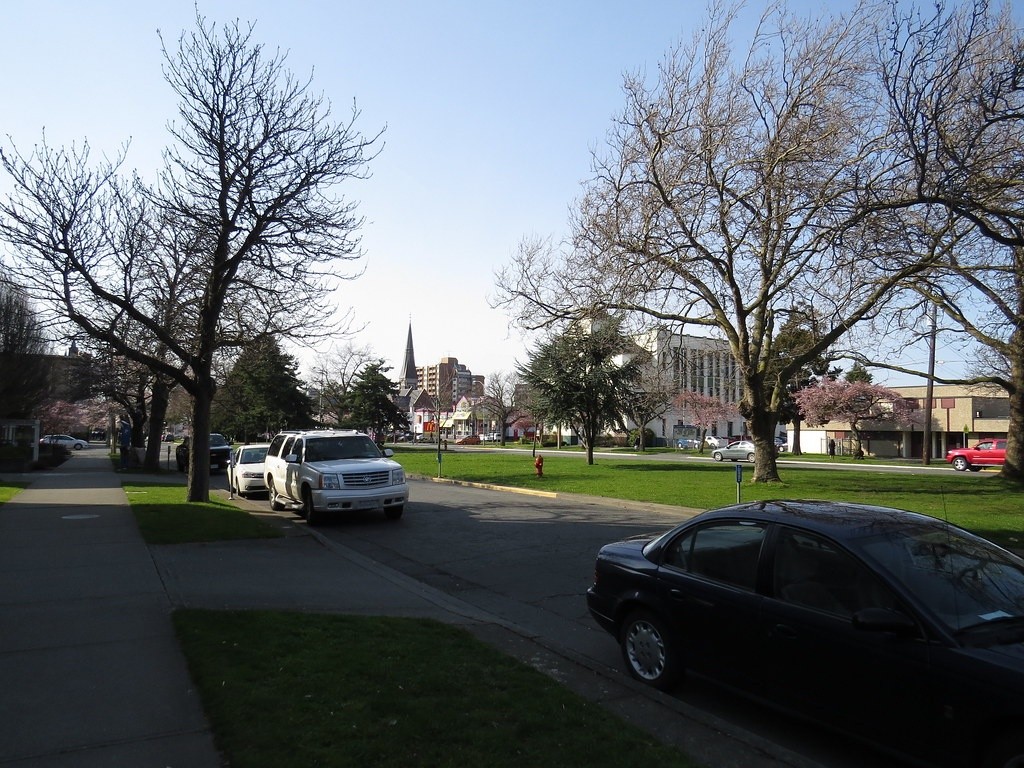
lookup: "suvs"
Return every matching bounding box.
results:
[263,427,409,526]
[948,439,1007,471]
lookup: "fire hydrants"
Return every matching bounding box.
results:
[533,454,543,476]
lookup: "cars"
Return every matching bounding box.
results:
[675,437,701,448]
[778,442,788,452]
[705,436,724,448]
[389,429,429,441]
[776,437,787,442]
[164,434,174,442]
[226,445,272,495]
[455,435,480,445]
[479,433,501,442]
[176,434,233,473]
[40,435,88,450]
[711,440,780,462]
[368,432,385,444]
[774,438,783,446]
[585,498,1024,768]
[161,434,165,441]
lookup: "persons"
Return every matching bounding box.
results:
[380,433,385,446]
[829,440,835,461]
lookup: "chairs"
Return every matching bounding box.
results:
[775,543,827,607]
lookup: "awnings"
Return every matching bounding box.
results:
[452,411,471,420]
[439,419,454,427]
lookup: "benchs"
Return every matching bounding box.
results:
[690,537,761,588]
